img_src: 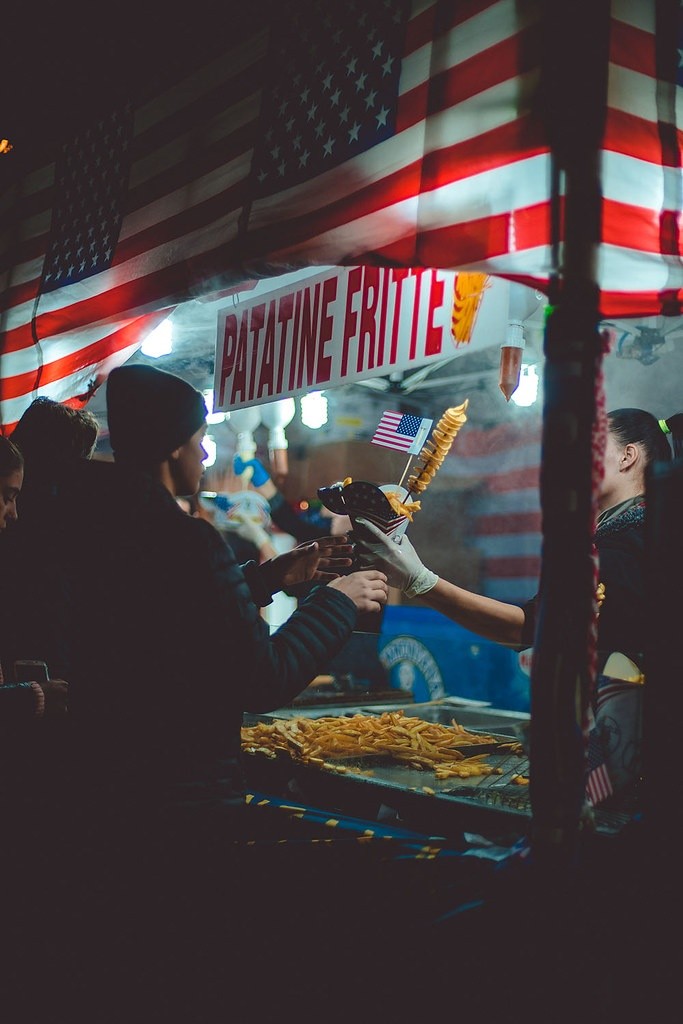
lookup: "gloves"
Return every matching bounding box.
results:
[346,518,438,598]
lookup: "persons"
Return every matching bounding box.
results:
[345,408,683,655]
[0,364,399,788]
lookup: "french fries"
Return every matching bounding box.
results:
[342,476,421,523]
[241,710,528,794]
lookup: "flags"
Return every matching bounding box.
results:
[369,410,433,456]
[585,734,614,808]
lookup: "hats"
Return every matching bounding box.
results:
[106,364,211,454]
